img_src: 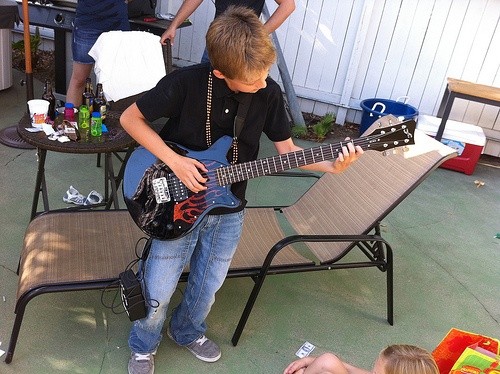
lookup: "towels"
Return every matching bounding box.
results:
[88,30,167,102]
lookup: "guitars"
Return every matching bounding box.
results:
[121,117,417,242]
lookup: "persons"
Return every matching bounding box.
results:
[119,6,442,374]
[160,0,296,63]
[67,0,130,110]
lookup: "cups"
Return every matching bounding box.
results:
[27,99,50,124]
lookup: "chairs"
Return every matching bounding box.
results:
[4,114,458,366]
[87,30,172,168]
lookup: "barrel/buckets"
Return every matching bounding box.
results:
[360,96,419,136]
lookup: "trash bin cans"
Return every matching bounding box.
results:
[359,96,420,136]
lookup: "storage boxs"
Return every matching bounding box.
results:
[416,114,486,176]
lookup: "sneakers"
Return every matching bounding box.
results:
[166,327,221,362]
[127,351,154,374]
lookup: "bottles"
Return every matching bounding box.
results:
[79,105,89,128]
[41,80,56,121]
[91,112,102,136]
[64,103,75,122]
[83,78,107,124]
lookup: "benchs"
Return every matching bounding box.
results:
[435,77,500,142]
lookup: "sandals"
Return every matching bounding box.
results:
[84,190,104,209]
[63,186,87,205]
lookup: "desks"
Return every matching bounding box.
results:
[16,106,141,275]
